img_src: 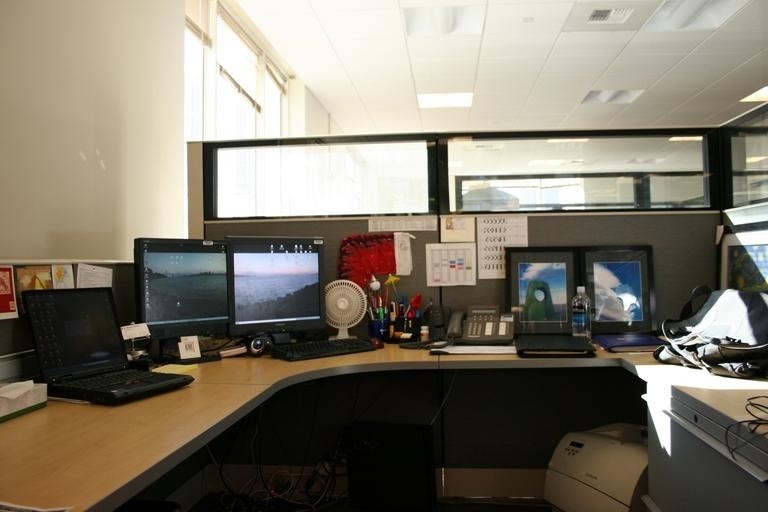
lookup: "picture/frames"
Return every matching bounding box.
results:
[505,246,580,340]
[715,220,768,292]
[579,245,659,336]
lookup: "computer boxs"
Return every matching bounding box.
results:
[344,420,437,512]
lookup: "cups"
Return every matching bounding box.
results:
[368,319,387,344]
[395,315,416,334]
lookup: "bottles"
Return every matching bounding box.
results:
[571,285,593,344]
[419,326,431,343]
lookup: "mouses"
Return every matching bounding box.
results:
[369,338,384,349]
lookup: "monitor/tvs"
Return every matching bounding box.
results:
[133,237,235,365]
[224,235,326,344]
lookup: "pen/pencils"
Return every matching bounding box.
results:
[366,294,411,322]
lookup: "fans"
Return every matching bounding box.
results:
[323,279,368,341]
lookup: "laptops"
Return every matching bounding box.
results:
[19,287,196,407]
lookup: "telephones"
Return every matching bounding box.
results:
[447,304,515,345]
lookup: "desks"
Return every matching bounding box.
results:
[0,339,768,512]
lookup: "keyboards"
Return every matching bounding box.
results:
[272,338,376,362]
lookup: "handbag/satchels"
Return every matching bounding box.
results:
[653,285,768,378]
